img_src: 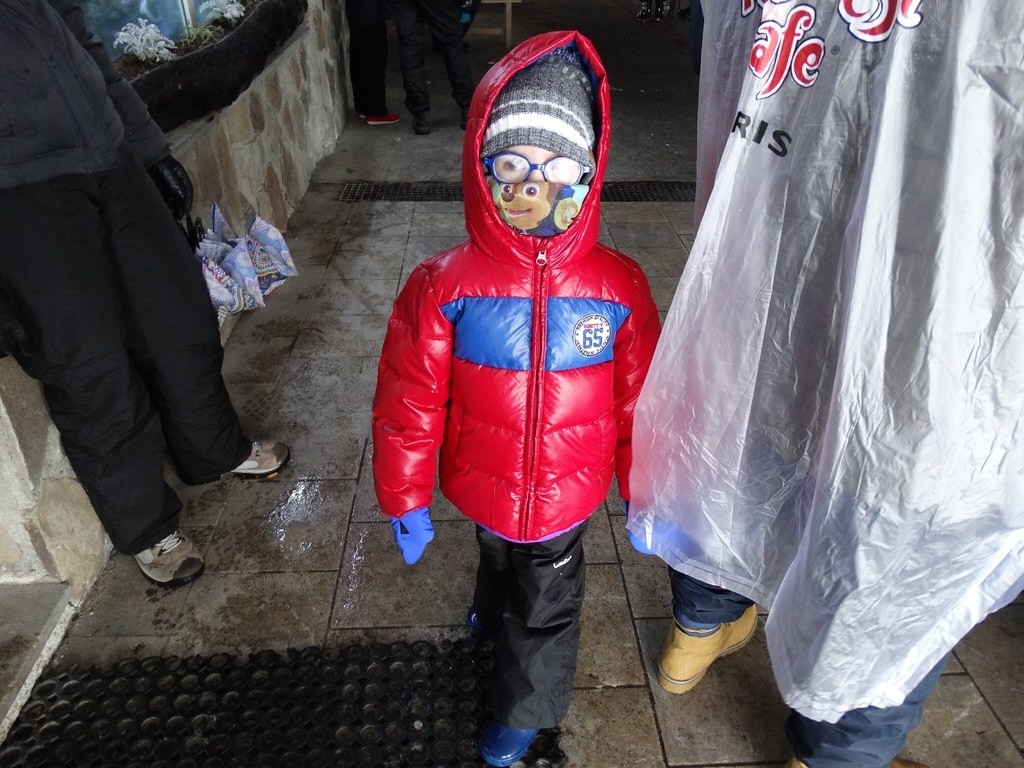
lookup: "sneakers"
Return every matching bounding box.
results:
[183,438,291,487]
[131,527,205,591]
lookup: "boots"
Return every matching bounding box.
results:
[655,0,664,23]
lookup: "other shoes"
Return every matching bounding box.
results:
[412,112,432,135]
[635,0,653,23]
[459,110,468,131]
[367,112,400,125]
[359,110,369,119]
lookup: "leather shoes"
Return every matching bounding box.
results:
[466,603,486,636]
[655,598,758,695]
[479,716,540,767]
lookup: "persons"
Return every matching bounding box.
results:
[388,0,487,134]
[345,0,402,125]
[647,0,1024,768]
[373,28,673,766]
[0,0,292,589]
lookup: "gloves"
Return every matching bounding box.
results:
[0,276,43,383]
[146,154,194,222]
[622,499,677,556]
[389,508,435,566]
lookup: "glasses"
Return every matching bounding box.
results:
[482,149,591,187]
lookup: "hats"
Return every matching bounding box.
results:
[479,50,597,186]
